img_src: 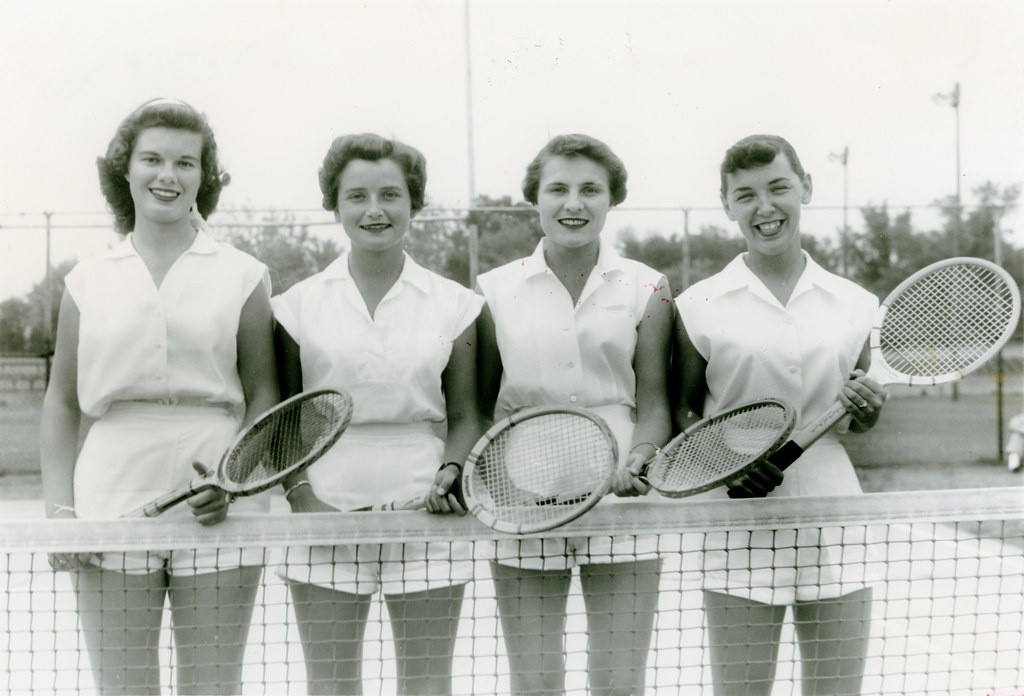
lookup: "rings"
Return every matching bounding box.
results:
[60,559,67,567]
[860,400,867,408]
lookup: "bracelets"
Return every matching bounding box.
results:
[630,442,659,455]
[285,481,311,498]
[439,461,462,472]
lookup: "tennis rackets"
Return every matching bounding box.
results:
[728,255,1020,501]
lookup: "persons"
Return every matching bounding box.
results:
[671,134,889,695]
[38,97,276,696]
[271,130,489,695]
[476,134,677,695]
